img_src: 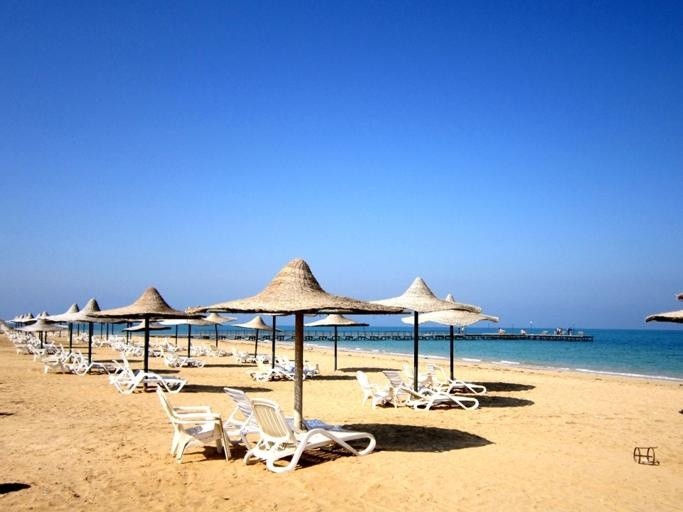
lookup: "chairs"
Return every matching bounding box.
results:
[221,387,339,435]
[243,397,375,474]
[67,329,319,382]
[152,382,233,463]
[5,330,188,395]
[349,359,487,412]
